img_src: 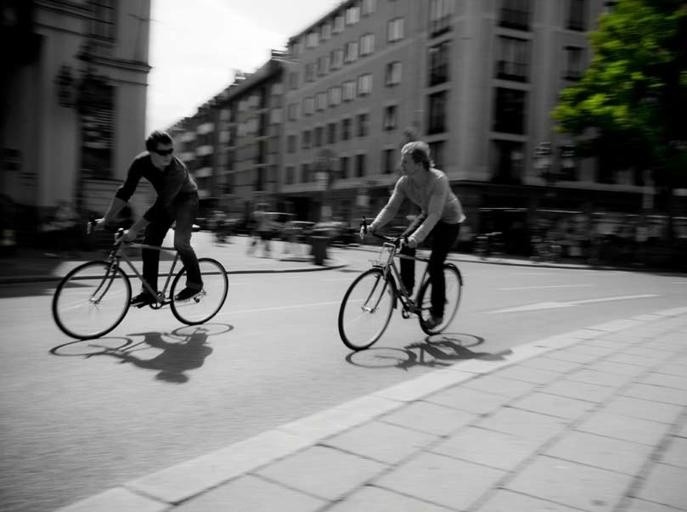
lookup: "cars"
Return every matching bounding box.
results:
[279,222,351,246]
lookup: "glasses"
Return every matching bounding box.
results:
[153,147,175,156]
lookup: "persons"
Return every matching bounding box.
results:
[42,201,78,253]
[213,210,226,242]
[91,128,205,306]
[249,201,290,255]
[359,141,466,328]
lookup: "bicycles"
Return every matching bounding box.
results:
[339,223,464,352]
[53,220,230,340]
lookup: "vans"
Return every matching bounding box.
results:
[246,212,294,233]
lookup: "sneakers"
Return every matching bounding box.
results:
[387,286,414,297]
[130,291,159,307]
[174,285,201,302]
[421,312,444,330]
